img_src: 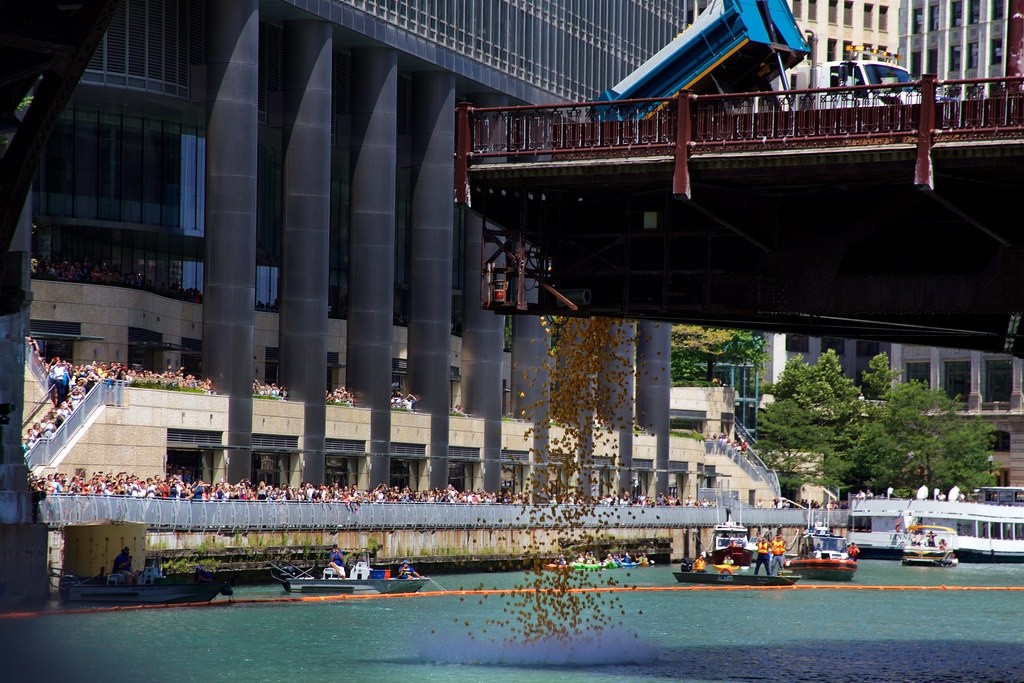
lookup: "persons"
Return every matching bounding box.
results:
[754,532,772,575]
[326,543,347,579]
[926,531,937,546]
[111,546,141,584]
[713,556,739,576]
[398,559,424,579]
[692,555,708,573]
[767,533,789,576]
[846,542,860,562]
[537,544,655,569]
[11,251,973,529]
[195,565,213,583]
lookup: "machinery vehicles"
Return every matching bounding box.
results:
[585,0,960,133]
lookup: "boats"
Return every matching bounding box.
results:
[272,560,432,597]
[778,528,857,582]
[615,561,637,568]
[600,562,616,569]
[636,560,649,567]
[901,524,960,567]
[843,486,1024,562]
[568,561,599,571]
[674,522,802,587]
[543,562,574,571]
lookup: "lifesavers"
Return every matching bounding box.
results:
[718,569,734,575]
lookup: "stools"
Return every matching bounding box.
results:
[107,573,135,585]
[325,567,345,580]
